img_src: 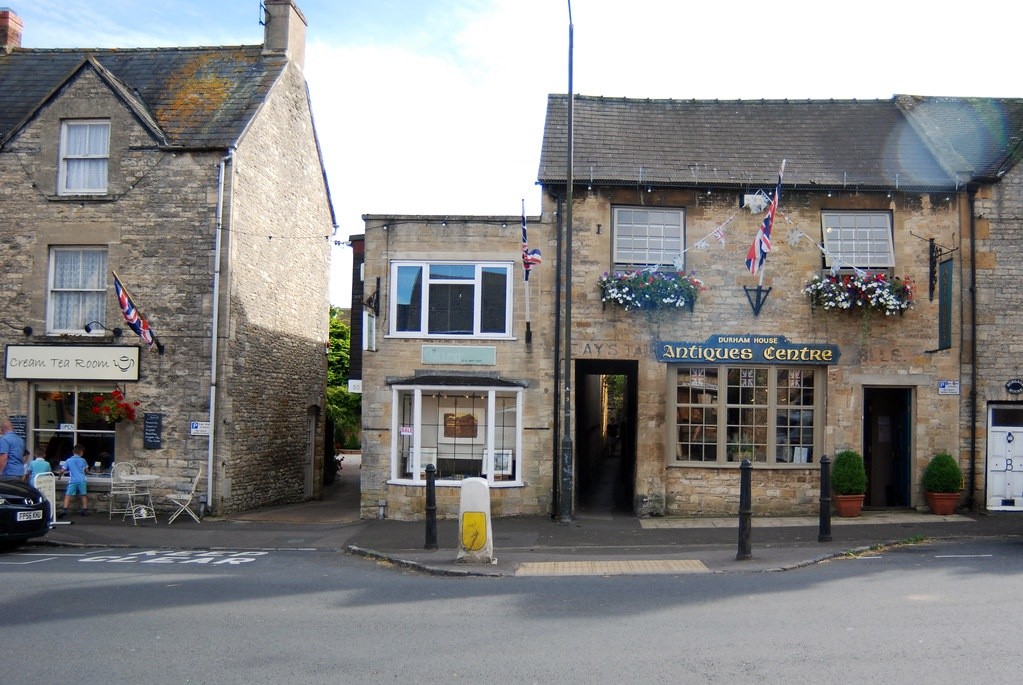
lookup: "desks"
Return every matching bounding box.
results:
[120,474,160,526]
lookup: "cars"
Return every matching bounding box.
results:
[0,475,50,545]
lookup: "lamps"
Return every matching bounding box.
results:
[84,321,122,336]
[741,193,770,209]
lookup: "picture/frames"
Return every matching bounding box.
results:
[407,446,438,474]
[481,448,512,475]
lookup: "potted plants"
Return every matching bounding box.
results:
[921,453,962,516]
[830,451,867,517]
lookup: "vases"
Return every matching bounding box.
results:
[103,416,114,423]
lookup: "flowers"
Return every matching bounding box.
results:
[597,263,708,347]
[802,270,918,365]
[91,389,139,424]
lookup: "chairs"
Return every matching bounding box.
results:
[110,462,137,521]
[165,469,202,524]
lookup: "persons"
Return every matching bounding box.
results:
[57,444,91,517]
[0,421,25,479]
[607,416,621,456]
[27,448,52,487]
[23,450,31,481]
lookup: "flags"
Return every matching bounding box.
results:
[744,179,781,275]
[758,193,866,278]
[521,216,542,270]
[115,280,155,350]
[648,227,727,275]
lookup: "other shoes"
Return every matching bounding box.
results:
[81,509,88,516]
[58,508,67,519]
